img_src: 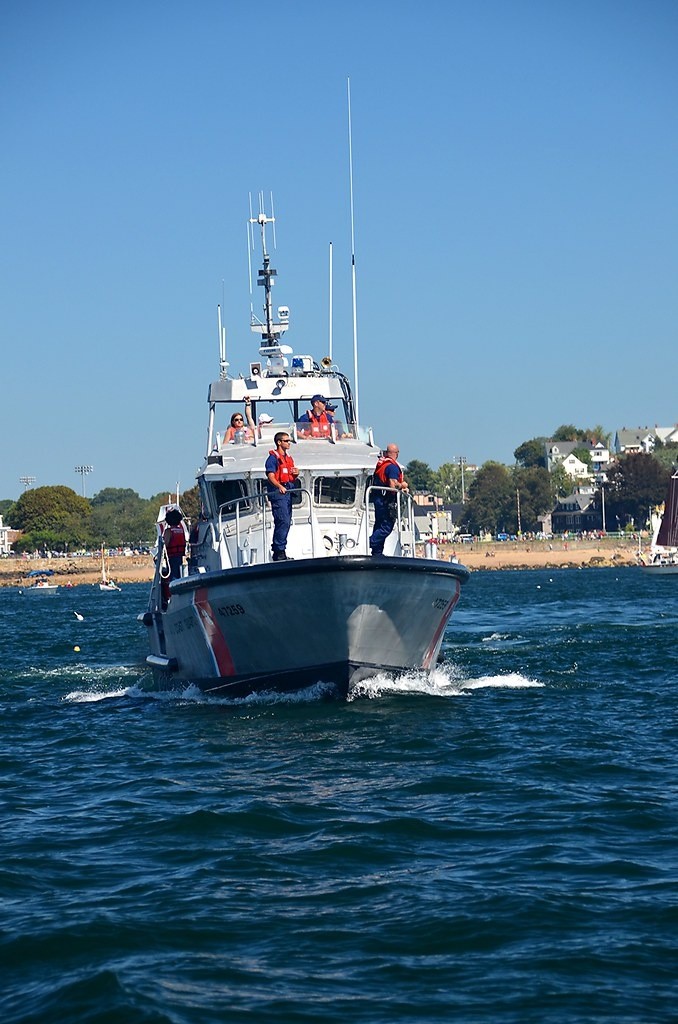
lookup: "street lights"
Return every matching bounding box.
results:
[74,465,94,498]
[20,476,37,491]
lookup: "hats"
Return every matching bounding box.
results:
[325,402,338,410]
[311,395,329,402]
[258,414,274,424]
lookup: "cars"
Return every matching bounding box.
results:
[496,533,517,542]
[0,546,150,558]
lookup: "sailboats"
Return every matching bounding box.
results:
[98,543,118,592]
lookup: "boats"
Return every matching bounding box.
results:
[133,78,469,713]
[26,569,59,596]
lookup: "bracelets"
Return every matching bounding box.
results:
[245,404,250,406]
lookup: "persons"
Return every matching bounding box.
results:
[296,394,353,440]
[163,509,186,579]
[243,395,275,439]
[265,432,299,561]
[428,529,606,565]
[223,413,249,444]
[369,444,408,557]
[632,534,637,540]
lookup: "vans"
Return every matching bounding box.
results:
[459,533,474,542]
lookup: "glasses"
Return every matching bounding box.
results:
[234,418,243,422]
[279,439,291,443]
